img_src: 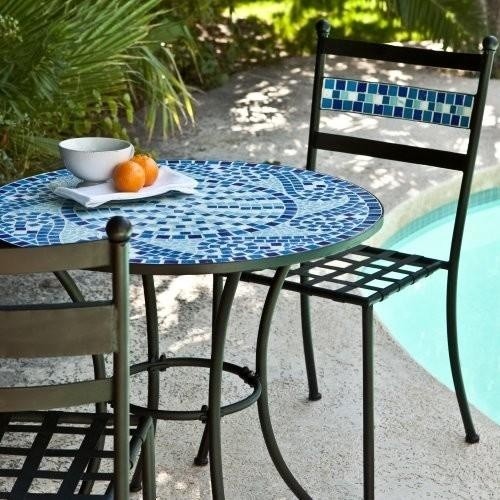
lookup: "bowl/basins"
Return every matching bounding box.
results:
[57,136,134,188]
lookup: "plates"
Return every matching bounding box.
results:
[55,164,198,209]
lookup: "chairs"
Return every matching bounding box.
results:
[0,216,158,499]
[193,19,500,500]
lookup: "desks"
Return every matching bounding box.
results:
[0,159,385,500]
